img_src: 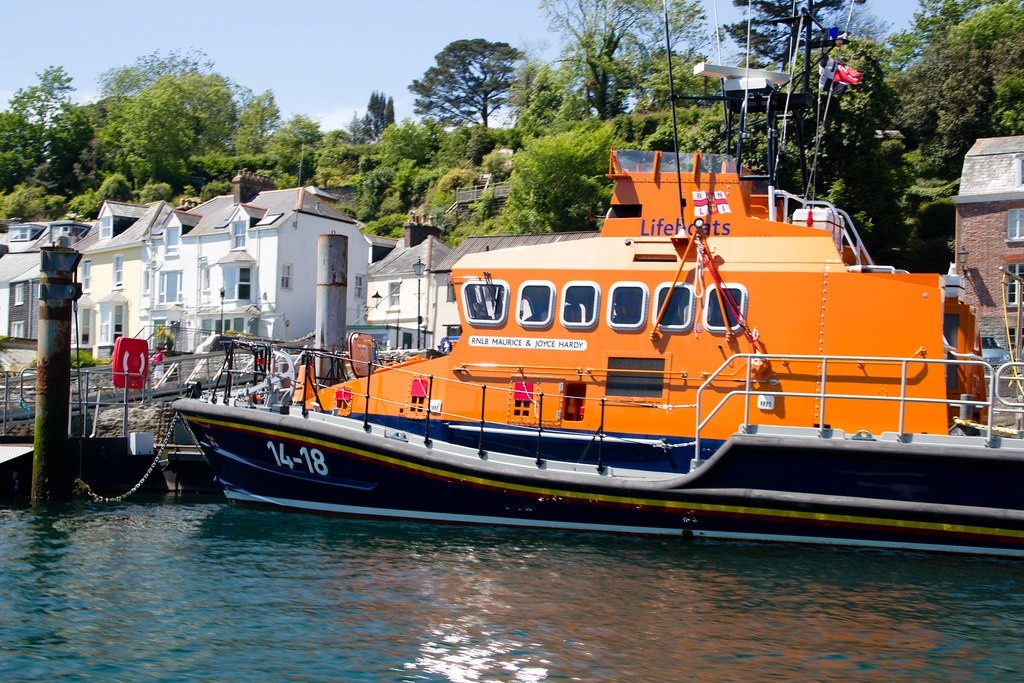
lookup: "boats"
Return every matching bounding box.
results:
[174,0,1024,558]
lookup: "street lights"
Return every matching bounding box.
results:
[413,255,426,349]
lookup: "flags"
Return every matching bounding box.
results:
[833,56,862,86]
[819,53,849,98]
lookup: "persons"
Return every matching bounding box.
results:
[153,347,164,387]
